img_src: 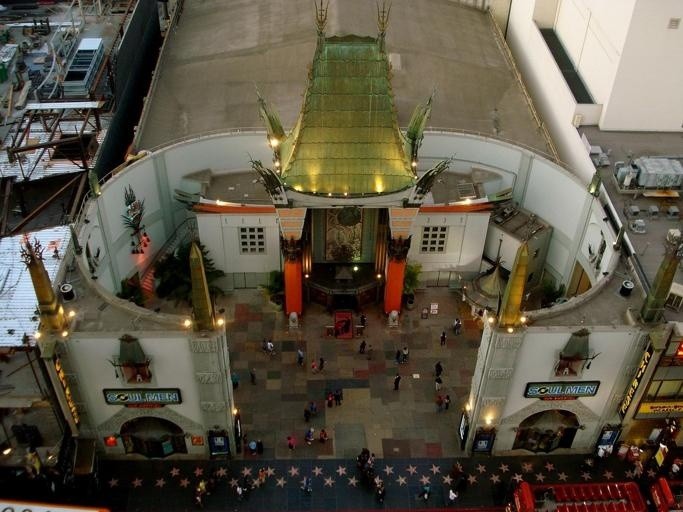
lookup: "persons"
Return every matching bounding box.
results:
[263,340,324,374]
[358,316,373,360]
[44,39,49,55]
[193,467,220,508]
[303,388,343,422]
[488,107,502,135]
[287,427,327,451]
[256,440,263,456]
[304,479,312,496]
[342,319,351,333]
[493,475,558,512]
[233,467,266,499]
[434,319,462,411]
[419,484,459,502]
[356,448,385,502]
[393,347,409,390]
[233,367,257,388]
